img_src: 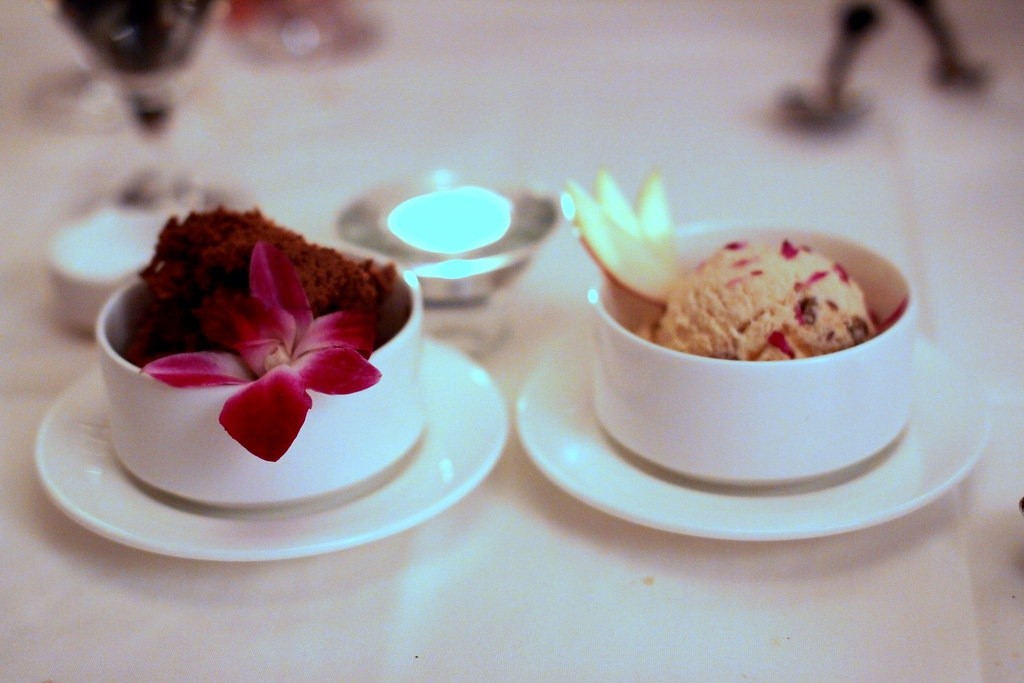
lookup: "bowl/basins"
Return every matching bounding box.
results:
[337,174,571,301]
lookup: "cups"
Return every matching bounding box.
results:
[48,210,170,333]
[588,223,918,485]
[94,269,426,508]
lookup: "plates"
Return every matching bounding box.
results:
[34,336,511,566]
[515,336,990,542]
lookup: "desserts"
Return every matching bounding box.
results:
[125,204,398,384]
[638,231,877,360]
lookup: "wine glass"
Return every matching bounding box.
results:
[53,0,229,210]
